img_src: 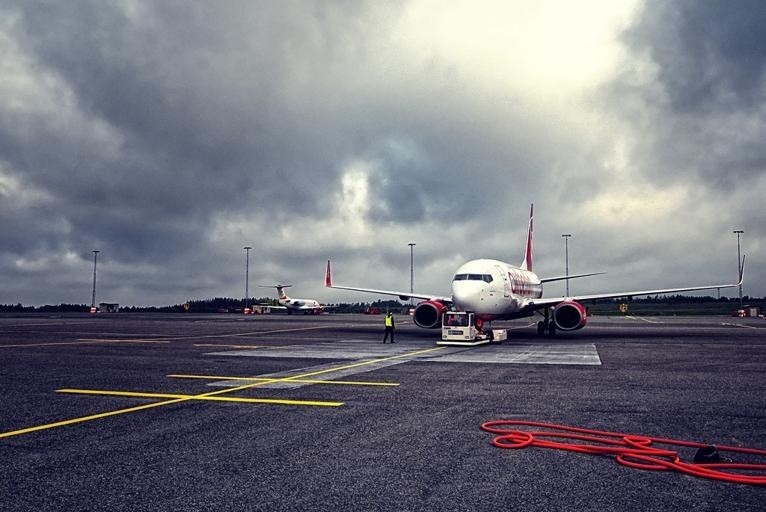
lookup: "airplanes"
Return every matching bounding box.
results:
[251,284,341,316]
[324,202,747,339]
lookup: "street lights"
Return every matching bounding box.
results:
[243,247,251,308]
[408,244,416,306]
[732,230,745,317]
[92,251,100,307]
[562,235,571,298]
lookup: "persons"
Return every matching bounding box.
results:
[383,310,398,343]
